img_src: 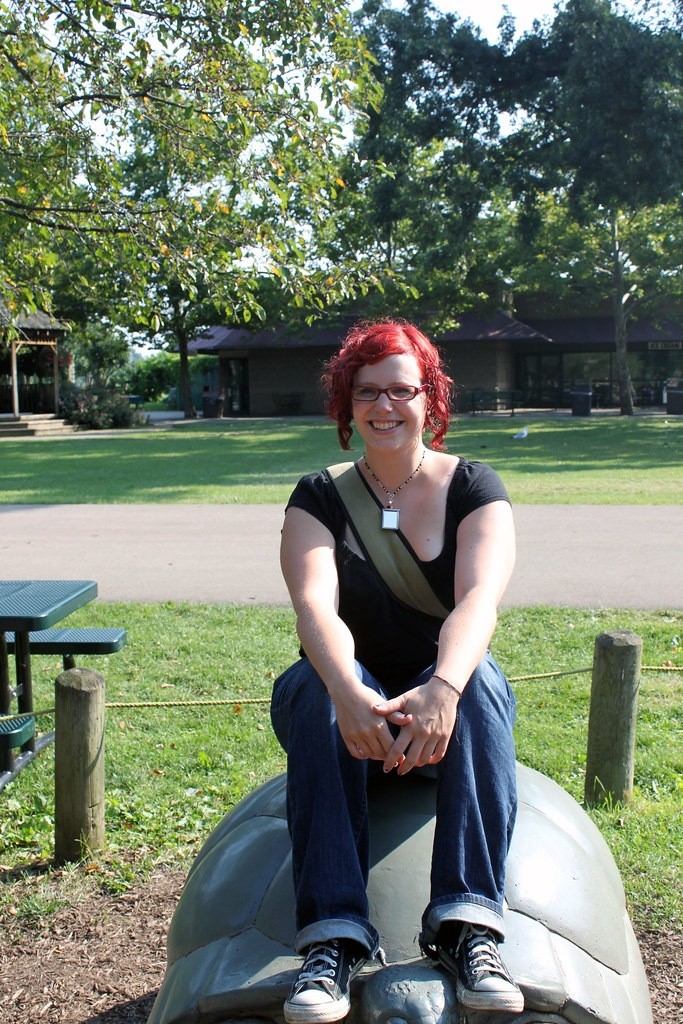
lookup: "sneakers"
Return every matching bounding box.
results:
[282,937,371,1024]
[430,921,525,1013]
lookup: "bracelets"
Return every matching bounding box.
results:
[429,675,462,698]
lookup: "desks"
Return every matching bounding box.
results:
[0,579,98,793]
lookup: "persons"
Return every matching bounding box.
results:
[271,319,523,1023]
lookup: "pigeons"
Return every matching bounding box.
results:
[510,427,528,439]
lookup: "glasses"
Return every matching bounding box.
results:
[347,383,432,402]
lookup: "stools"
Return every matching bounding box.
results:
[0,714,35,749]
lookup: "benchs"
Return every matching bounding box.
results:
[466,390,516,417]
[0,628,126,671]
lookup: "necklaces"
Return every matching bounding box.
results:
[363,445,426,530]
